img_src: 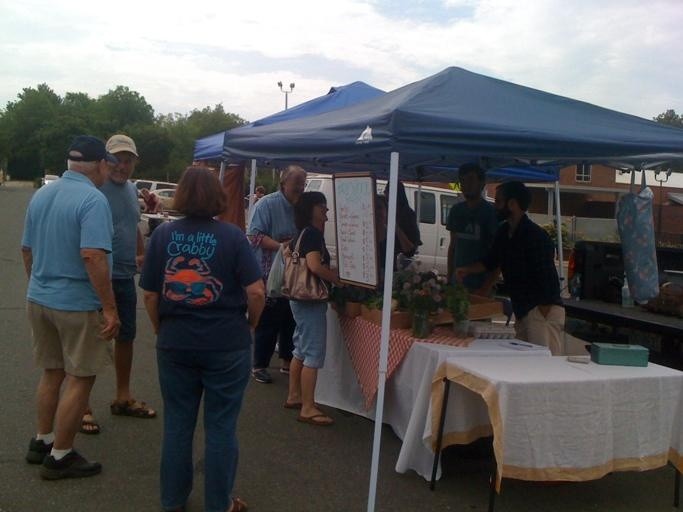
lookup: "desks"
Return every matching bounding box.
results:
[563,298,683,340]
[314,301,552,483]
[142,213,180,239]
[422,355,683,512]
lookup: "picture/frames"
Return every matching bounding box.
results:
[332,171,381,291]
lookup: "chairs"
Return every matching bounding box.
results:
[495,297,514,326]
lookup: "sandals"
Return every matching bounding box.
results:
[227,497,247,512]
[111,400,155,418]
[80,408,99,434]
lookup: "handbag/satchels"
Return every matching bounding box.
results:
[281,254,330,302]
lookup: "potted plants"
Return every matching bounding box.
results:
[328,284,409,329]
[449,278,472,339]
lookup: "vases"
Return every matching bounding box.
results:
[412,308,429,339]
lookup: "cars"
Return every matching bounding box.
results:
[42,174,59,185]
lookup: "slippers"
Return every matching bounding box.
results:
[284,400,336,425]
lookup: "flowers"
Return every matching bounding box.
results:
[400,260,449,335]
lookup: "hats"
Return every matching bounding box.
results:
[68,135,139,162]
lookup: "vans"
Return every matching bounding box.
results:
[301,173,494,278]
[128,178,178,211]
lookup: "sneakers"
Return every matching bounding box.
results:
[26,437,101,480]
[251,368,273,383]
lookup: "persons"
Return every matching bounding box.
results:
[137,165,266,512]
[455,180,566,355]
[21,135,121,480]
[379,180,423,272]
[284,191,348,426]
[248,165,308,383]
[79,135,158,435]
[445,163,504,321]
[140,188,162,213]
[253,185,268,205]
